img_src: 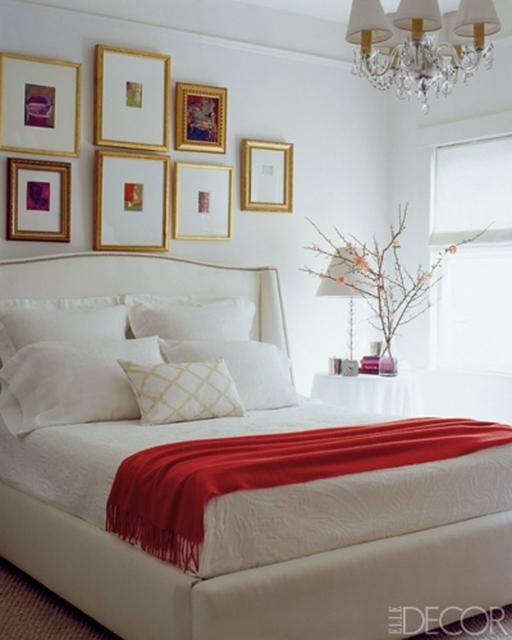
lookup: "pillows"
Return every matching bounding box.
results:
[161,339,301,414]
[116,288,257,349]
[116,355,246,430]
[0,333,170,443]
[0,294,131,362]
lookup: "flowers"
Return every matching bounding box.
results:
[295,200,499,371]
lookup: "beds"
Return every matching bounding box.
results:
[0,256,512,637]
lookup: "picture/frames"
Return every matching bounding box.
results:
[168,159,237,244]
[172,79,230,157]
[92,147,171,254]
[90,42,175,158]
[3,154,74,246]
[0,50,85,163]
[237,134,296,215]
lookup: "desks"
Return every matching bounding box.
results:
[309,370,417,420]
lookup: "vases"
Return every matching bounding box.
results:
[377,334,400,377]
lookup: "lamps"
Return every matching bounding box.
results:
[314,246,386,362]
[342,0,504,109]
